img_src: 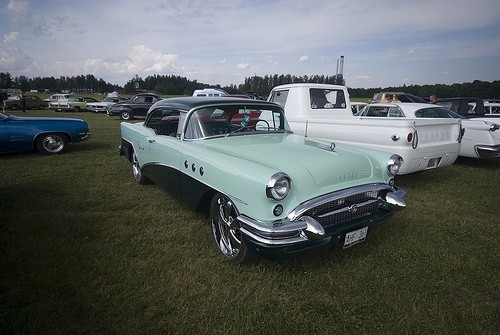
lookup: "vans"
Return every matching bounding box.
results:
[192,88,230,97]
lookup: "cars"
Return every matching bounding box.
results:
[352,91,500,114]
[108,92,182,121]
[354,102,500,159]
[0,111,91,156]
[162,92,264,126]
[0,89,127,114]
[118,97,408,265]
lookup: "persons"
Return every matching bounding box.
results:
[20,93,25,112]
[386,95,393,102]
[427,94,438,104]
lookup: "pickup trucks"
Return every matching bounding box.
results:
[256,83,466,176]
